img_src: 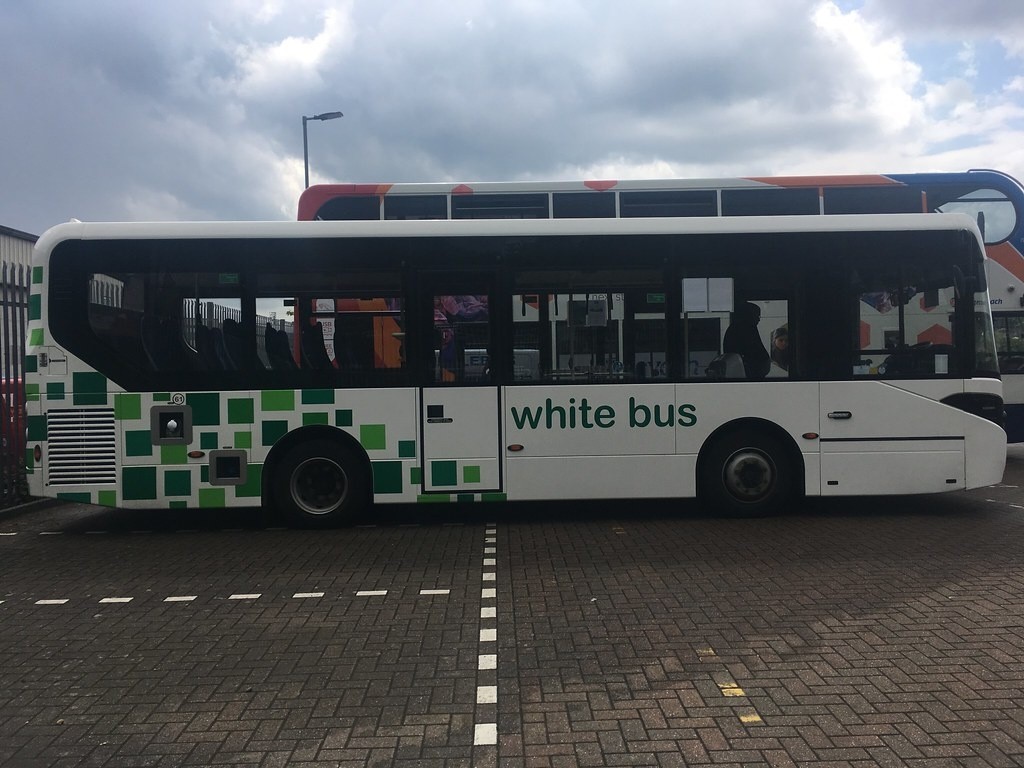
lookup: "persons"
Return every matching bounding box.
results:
[769,328,789,371]
[723,303,772,378]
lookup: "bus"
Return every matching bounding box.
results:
[20,215,1012,536]
[291,168,1024,445]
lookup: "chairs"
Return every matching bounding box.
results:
[707,351,750,377]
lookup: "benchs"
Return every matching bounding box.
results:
[196,325,238,387]
[224,318,270,389]
[300,322,339,387]
[139,314,195,391]
[90,304,144,368]
[334,321,361,386]
[265,324,309,387]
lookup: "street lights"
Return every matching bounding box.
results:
[302,111,345,187]
